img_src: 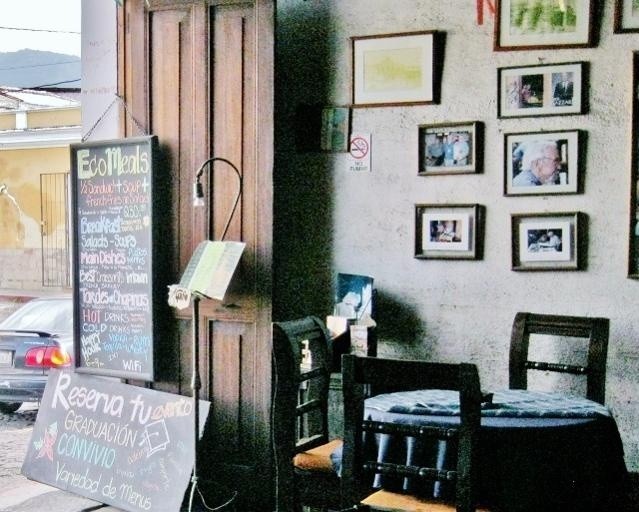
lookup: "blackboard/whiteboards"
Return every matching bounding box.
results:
[67,135,157,385]
[18,365,215,510]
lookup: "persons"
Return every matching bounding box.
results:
[513,142,525,179]
[429,138,443,164]
[436,221,459,242]
[537,231,559,248]
[554,72,573,100]
[441,135,454,166]
[513,141,561,186]
[527,84,531,90]
[453,134,470,165]
[522,83,539,103]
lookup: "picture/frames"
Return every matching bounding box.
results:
[495,60,592,118]
[627,50,639,282]
[614,0,639,33]
[502,128,588,195]
[512,213,584,272]
[349,31,446,107]
[414,201,485,261]
[294,104,352,154]
[417,121,485,174]
[492,0,600,53]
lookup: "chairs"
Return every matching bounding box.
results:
[266,314,344,512]
[336,356,484,511]
[507,311,612,406]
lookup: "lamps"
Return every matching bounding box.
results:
[187,158,242,512]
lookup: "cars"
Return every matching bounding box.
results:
[0,295,74,415]
